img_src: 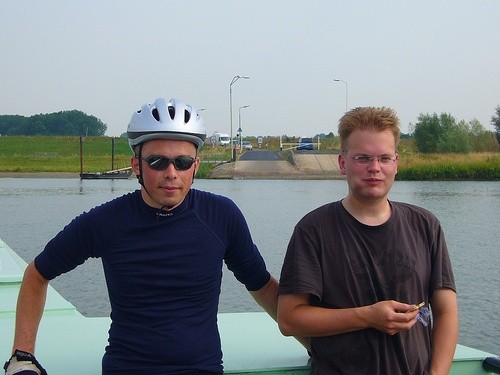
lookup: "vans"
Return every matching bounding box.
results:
[212,133,231,148]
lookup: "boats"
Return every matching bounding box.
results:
[80,166,133,179]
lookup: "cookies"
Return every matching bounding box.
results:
[406,301,425,312]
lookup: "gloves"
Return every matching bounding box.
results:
[3,350,48,375]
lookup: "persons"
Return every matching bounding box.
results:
[2,96,315,375]
[277,106,458,375]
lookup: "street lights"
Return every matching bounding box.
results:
[333,78,348,114]
[237,105,250,155]
[229,74,250,162]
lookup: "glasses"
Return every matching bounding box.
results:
[341,151,395,168]
[134,153,196,171]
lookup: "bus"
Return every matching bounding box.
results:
[298,136,314,150]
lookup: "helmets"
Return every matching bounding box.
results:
[126,98,207,152]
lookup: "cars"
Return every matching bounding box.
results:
[241,141,253,151]
[230,140,239,148]
